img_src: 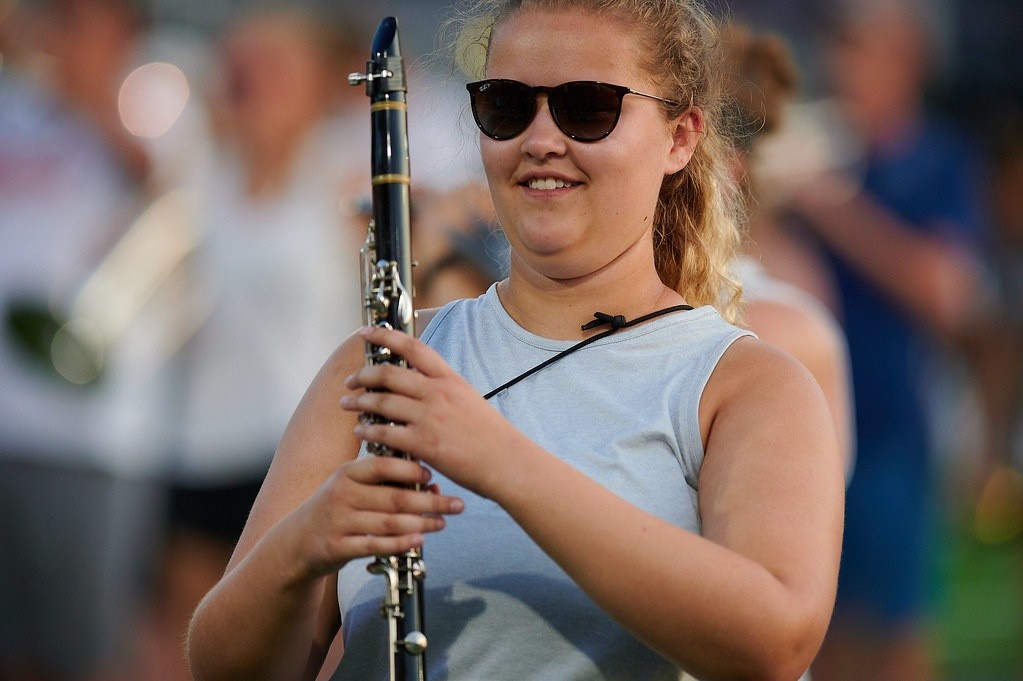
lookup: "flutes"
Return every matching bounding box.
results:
[342,11,436,681]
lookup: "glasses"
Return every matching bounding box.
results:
[466,77,680,143]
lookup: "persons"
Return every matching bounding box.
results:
[184,0,846,680]
[0,0,1000,681]
